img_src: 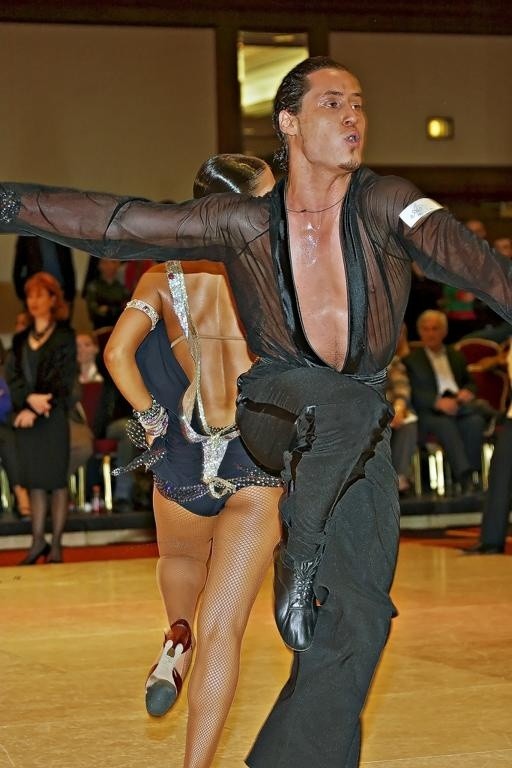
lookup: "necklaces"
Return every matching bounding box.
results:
[285,196,345,213]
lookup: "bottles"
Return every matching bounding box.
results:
[91,485,109,513]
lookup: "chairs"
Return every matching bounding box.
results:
[414,338,512,498]
[0,326,116,511]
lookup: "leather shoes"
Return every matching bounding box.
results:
[463,541,507,555]
[271,541,323,652]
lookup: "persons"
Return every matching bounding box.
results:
[1,199,176,565]
[383,221,512,554]
[104,150,287,767]
[0,56,512,768]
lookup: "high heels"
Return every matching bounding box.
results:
[143,619,198,719]
[47,557,63,564]
[15,542,51,566]
